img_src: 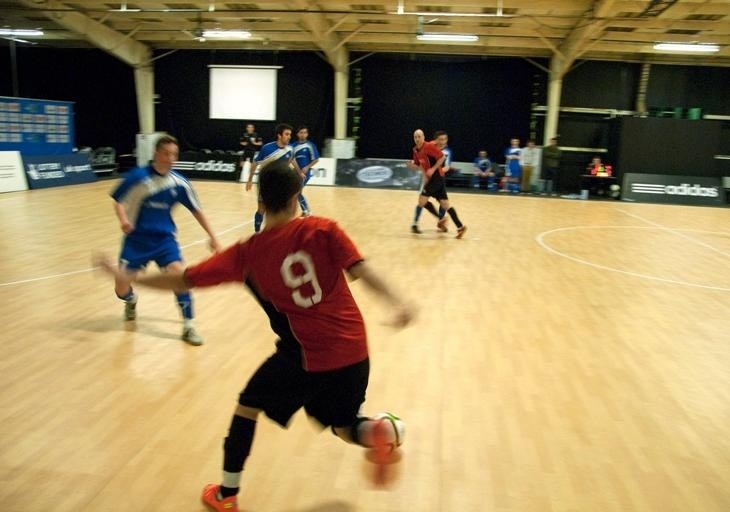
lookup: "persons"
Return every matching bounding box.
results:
[411,130,451,233]
[110,137,222,346]
[471,136,562,195]
[239,123,263,192]
[405,129,467,240]
[93,164,415,511]
[291,125,319,218]
[245,122,306,234]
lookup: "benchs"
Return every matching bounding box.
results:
[445,172,507,189]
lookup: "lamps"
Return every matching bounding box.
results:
[201,29,251,39]
[653,42,720,53]
[416,34,479,42]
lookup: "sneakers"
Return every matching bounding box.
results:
[126,294,137,320]
[183,326,201,345]
[457,226,466,238]
[437,217,448,232]
[204,484,240,512]
[413,224,420,233]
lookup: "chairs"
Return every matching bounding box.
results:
[78,147,120,175]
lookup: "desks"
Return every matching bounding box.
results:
[579,175,620,200]
[519,165,533,192]
